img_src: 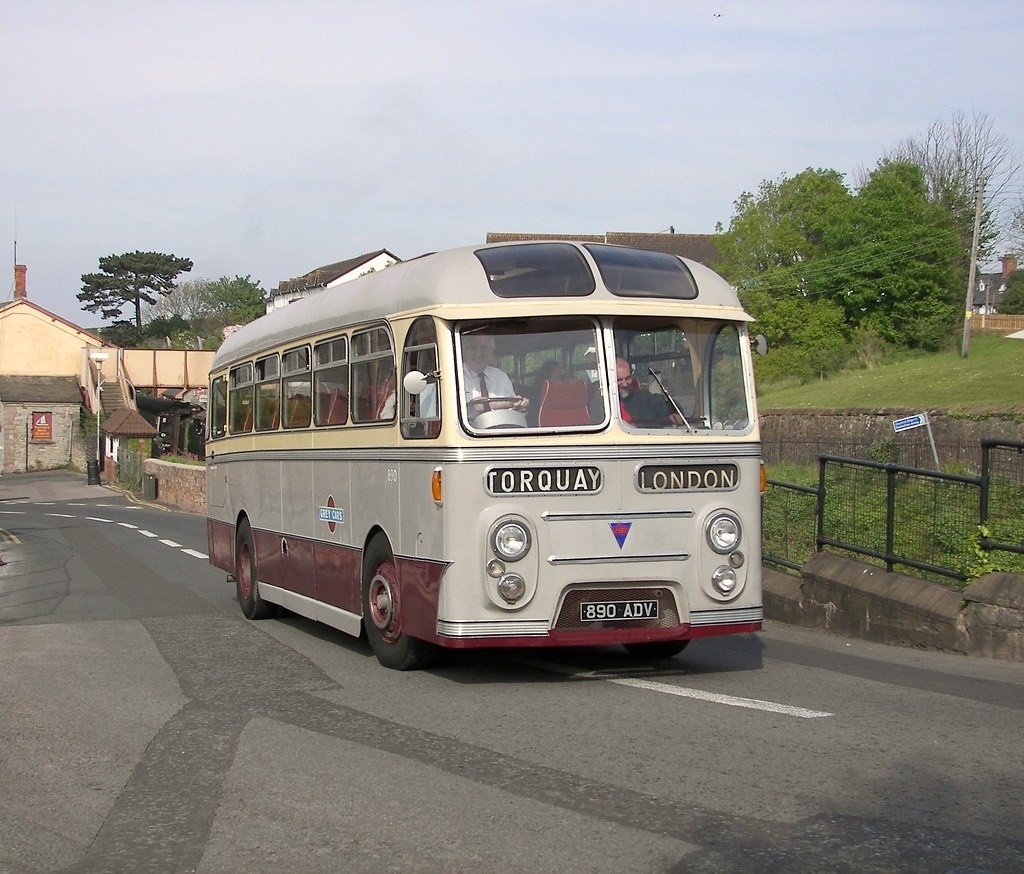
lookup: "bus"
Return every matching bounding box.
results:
[205,238,768,673]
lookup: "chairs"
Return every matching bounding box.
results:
[223,377,594,427]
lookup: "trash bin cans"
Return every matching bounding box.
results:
[86,459,101,485]
[142,472,156,501]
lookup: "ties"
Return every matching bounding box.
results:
[477,373,491,412]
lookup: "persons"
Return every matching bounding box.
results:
[378,332,604,439]
[616,357,684,427]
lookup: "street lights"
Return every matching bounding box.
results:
[89,353,110,468]
